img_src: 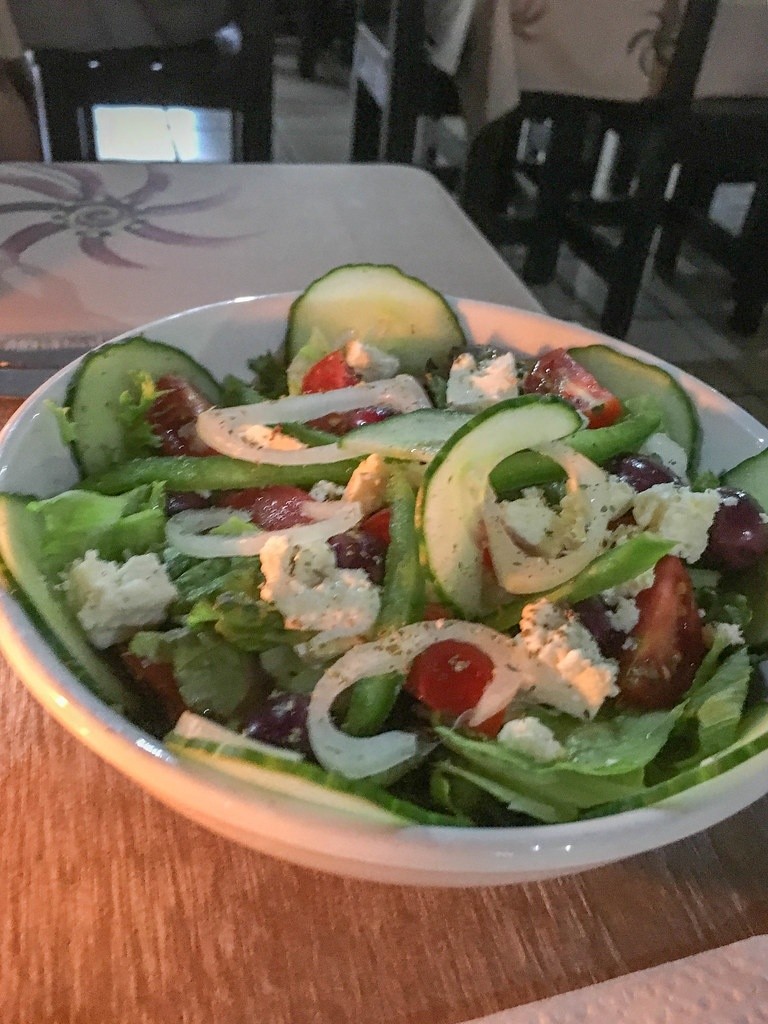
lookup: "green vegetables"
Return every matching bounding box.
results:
[22,417,748,823]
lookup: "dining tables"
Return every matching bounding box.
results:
[0,163,768,1024]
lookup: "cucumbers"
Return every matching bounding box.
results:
[526,346,702,482]
[581,697,768,820]
[716,446,768,515]
[0,492,142,719]
[283,262,466,390]
[61,337,220,484]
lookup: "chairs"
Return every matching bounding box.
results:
[33,0,768,340]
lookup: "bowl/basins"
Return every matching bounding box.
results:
[1,289,768,888]
